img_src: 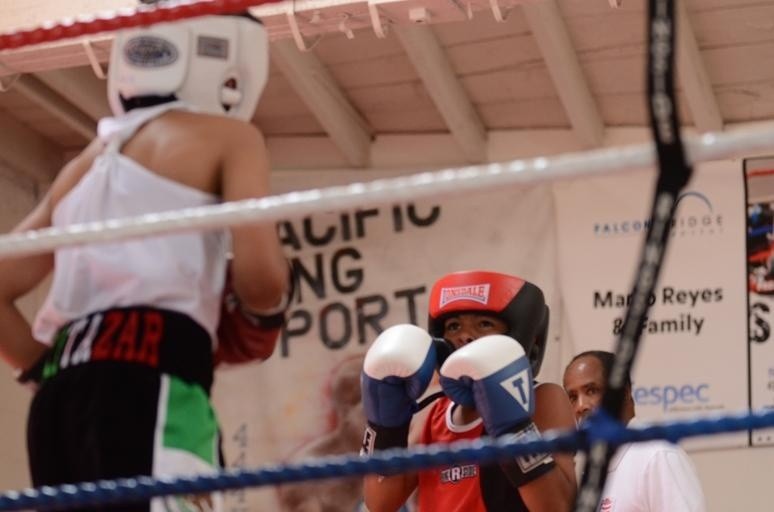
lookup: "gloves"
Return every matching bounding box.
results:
[216,257,289,370]
[359,323,438,455]
[439,334,556,487]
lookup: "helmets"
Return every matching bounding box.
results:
[427,270,550,378]
[108,0,269,123]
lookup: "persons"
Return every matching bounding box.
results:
[561,351,705,512]
[358,270,578,511]
[0,0,295,511]
